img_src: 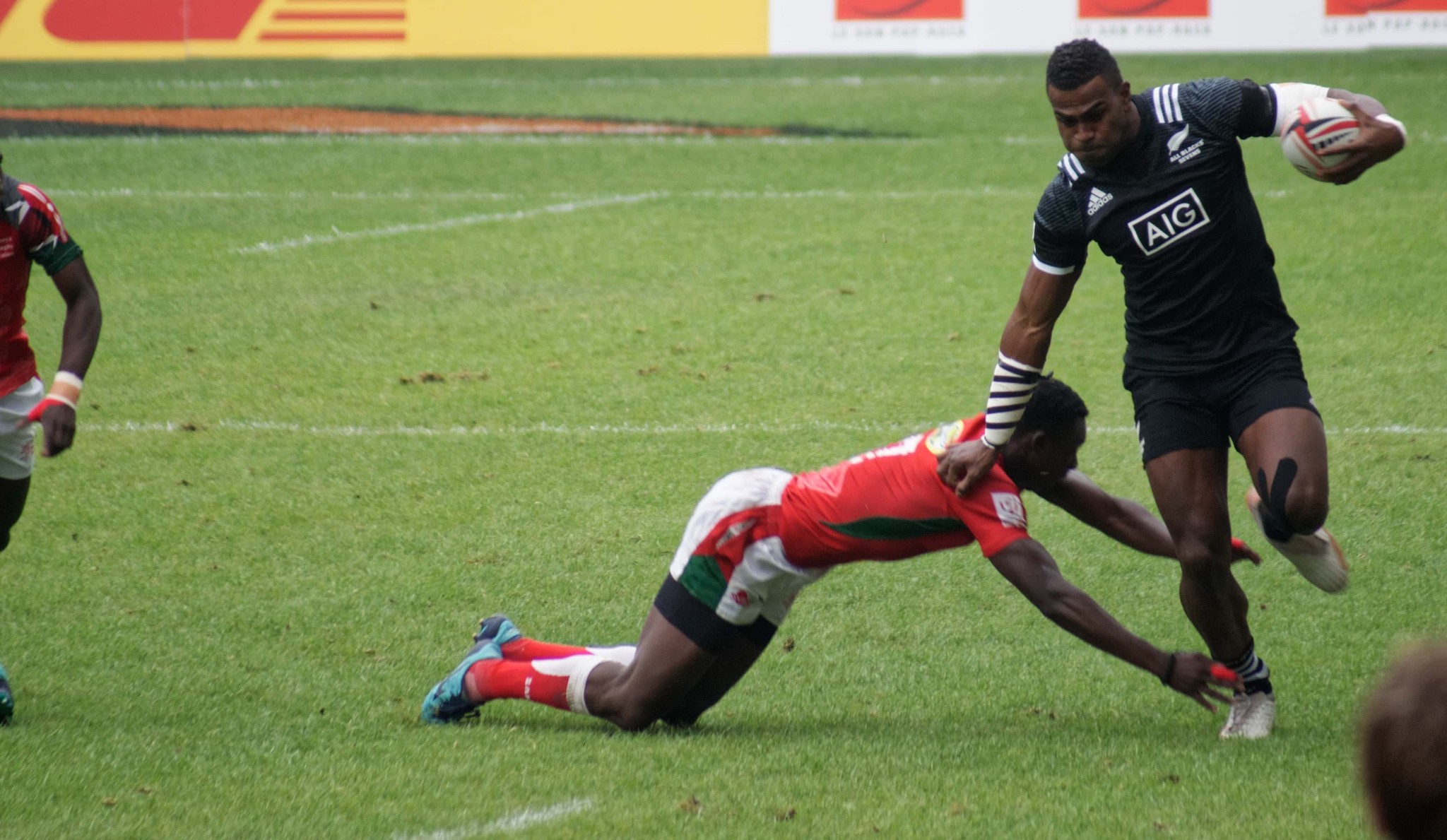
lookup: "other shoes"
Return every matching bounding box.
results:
[1,671,15,721]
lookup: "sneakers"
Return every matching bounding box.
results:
[470,614,522,648]
[422,638,503,723]
[1218,692,1281,742]
[1245,485,1351,594]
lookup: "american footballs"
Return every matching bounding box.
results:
[1279,95,1362,184]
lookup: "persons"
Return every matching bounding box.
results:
[937,40,1406,744]
[1357,636,1447,840]
[0,150,101,726]
[420,371,1262,735]
